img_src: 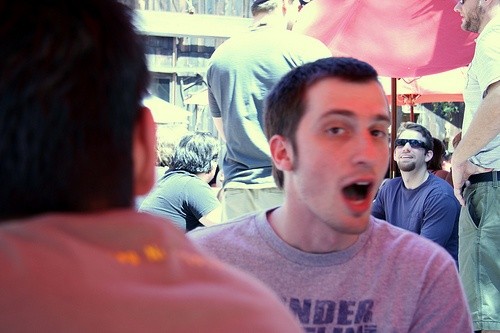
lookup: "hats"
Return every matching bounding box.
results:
[255,0,309,6]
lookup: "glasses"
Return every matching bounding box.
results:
[394,138,427,149]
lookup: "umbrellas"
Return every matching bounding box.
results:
[294,0,479,178]
[380,66,470,123]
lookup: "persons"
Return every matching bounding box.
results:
[207,0,332,222]
[184,57,474,333]
[372,122,462,267]
[452,0,500,333]
[0,0,302,333]
[427,138,452,185]
[138,135,224,234]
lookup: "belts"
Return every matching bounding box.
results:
[469,171,500,184]
